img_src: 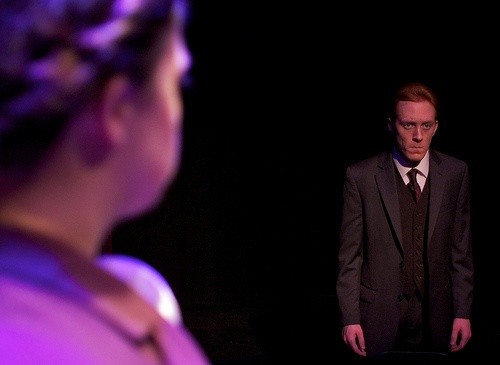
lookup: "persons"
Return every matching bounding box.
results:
[338,85,473,365]
[0,0,209,365]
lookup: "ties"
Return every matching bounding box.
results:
[406,168,421,205]
